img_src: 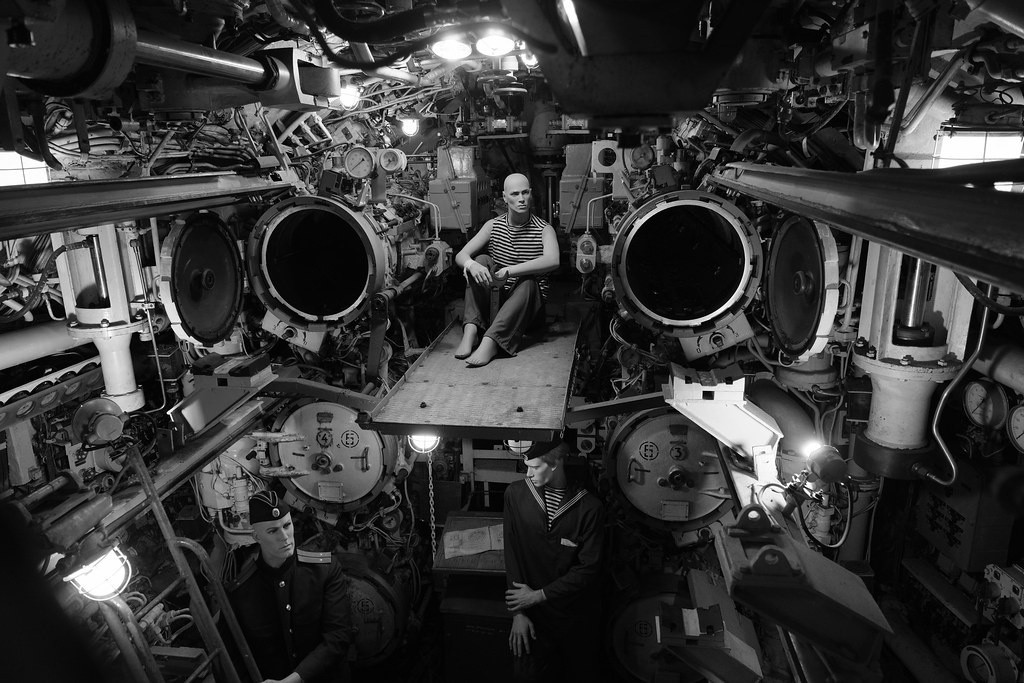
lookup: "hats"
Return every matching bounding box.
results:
[522,432,561,460]
[246,489,290,525]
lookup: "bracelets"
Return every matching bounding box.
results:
[463,260,476,283]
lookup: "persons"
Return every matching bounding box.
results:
[456,173,560,367]
[504,433,605,683]
[212,491,354,683]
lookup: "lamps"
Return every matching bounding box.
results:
[407,435,441,453]
[62,543,132,601]
[476,28,515,57]
[398,111,423,138]
[340,85,362,111]
[432,33,472,59]
[502,440,536,456]
[806,445,847,483]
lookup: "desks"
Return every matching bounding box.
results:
[432,516,507,577]
